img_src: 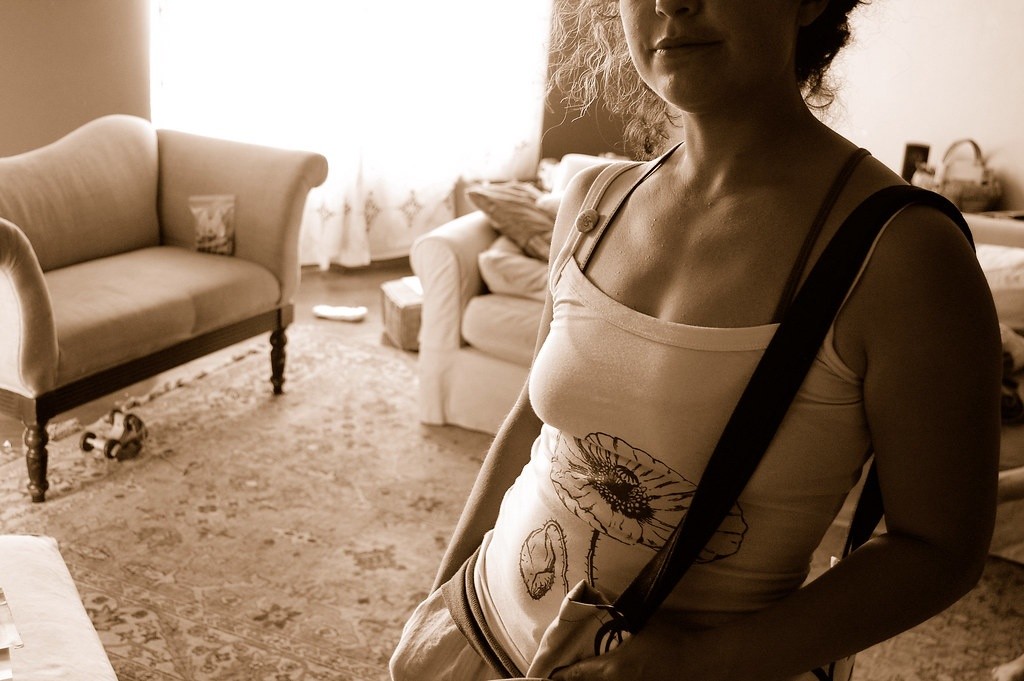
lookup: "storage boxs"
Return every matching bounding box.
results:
[381,276,422,349]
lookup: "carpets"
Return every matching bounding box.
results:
[0,332,1024,681]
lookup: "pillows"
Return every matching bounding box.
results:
[476,234,548,302]
[467,181,555,260]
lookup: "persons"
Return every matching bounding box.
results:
[385,0,1001,681]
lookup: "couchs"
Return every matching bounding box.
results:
[407,151,1024,565]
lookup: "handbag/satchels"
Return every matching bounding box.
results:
[522,580,857,681]
[912,137,1002,212]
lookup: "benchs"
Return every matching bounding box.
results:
[0,112,329,502]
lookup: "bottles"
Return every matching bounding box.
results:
[910,165,936,190]
